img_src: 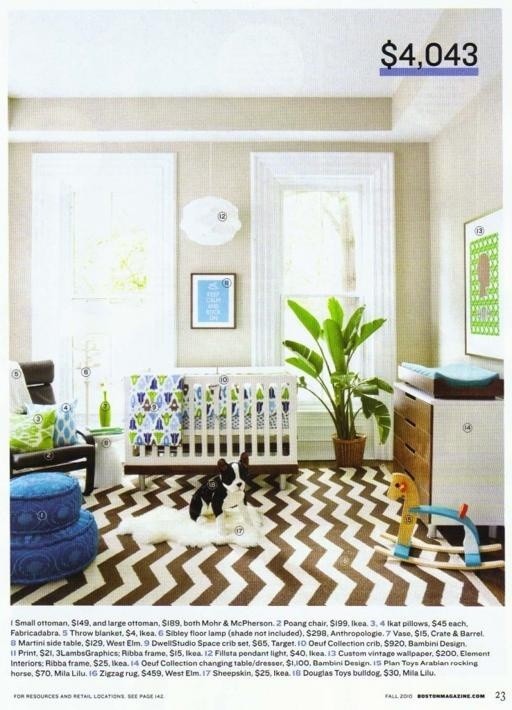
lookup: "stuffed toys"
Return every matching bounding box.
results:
[189,452,254,536]
[373,471,504,571]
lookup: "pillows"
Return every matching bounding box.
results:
[10,410,56,454]
[9,360,33,413]
[25,399,80,448]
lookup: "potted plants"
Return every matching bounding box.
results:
[283,297,394,467]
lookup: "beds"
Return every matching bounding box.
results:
[119,366,298,491]
[93,433,125,488]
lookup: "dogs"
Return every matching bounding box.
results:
[187,449,265,538]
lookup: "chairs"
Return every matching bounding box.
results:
[10,360,95,497]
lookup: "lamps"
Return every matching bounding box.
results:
[180,195,241,248]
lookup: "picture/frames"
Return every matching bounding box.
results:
[463,204,503,360]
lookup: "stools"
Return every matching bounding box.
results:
[10,509,99,586]
[10,472,82,536]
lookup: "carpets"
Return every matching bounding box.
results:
[11,463,503,607]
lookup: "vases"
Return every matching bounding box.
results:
[99,391,111,427]
